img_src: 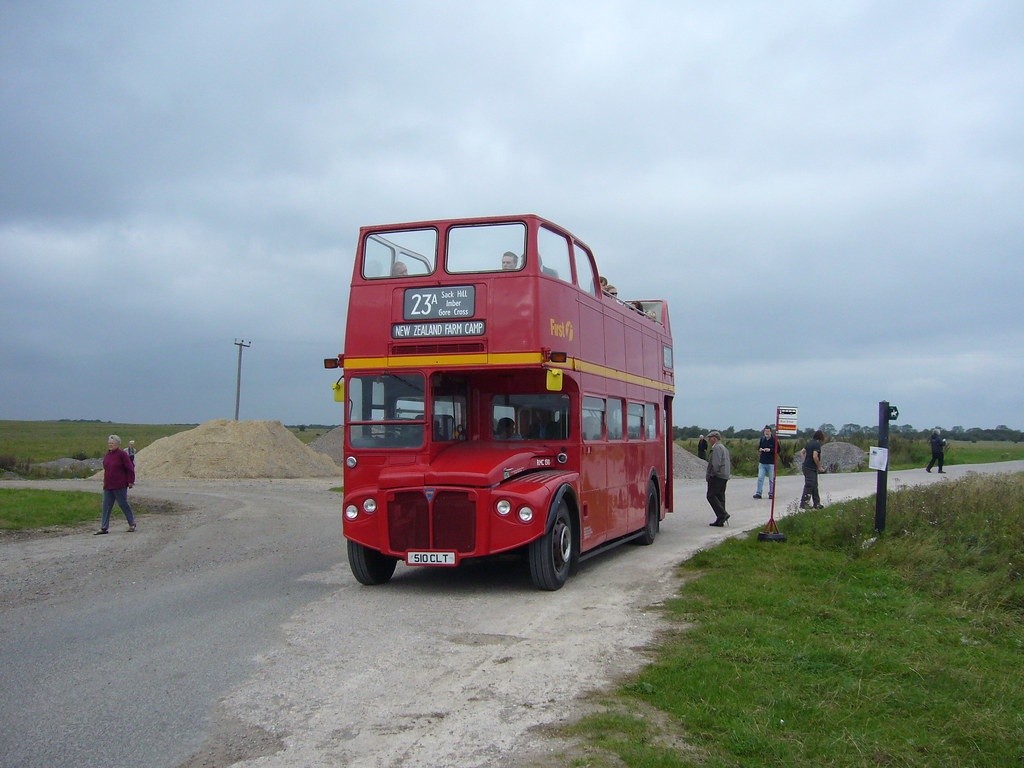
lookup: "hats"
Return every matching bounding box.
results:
[706,431,721,440]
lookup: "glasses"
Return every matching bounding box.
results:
[610,292,617,296]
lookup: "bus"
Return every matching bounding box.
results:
[322,214,676,593]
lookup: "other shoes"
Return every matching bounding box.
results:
[127,524,136,532]
[709,520,723,527]
[925,468,931,473]
[768,495,773,499]
[94,529,109,535]
[799,503,811,509]
[938,471,946,473]
[719,515,730,524]
[752,494,762,498]
[813,502,824,508]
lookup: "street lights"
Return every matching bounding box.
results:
[233,337,252,420]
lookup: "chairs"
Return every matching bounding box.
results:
[416,414,455,441]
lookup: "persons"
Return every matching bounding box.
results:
[502,251,518,270]
[705,431,731,527]
[391,262,408,276]
[521,253,557,278]
[606,286,620,300]
[643,309,658,323]
[92,435,137,536]
[628,301,642,316]
[753,425,781,499]
[798,430,825,510]
[591,277,607,299]
[697,434,707,459]
[123,440,136,489]
[492,416,522,441]
[925,429,946,474]
[582,416,600,441]
[524,402,561,440]
